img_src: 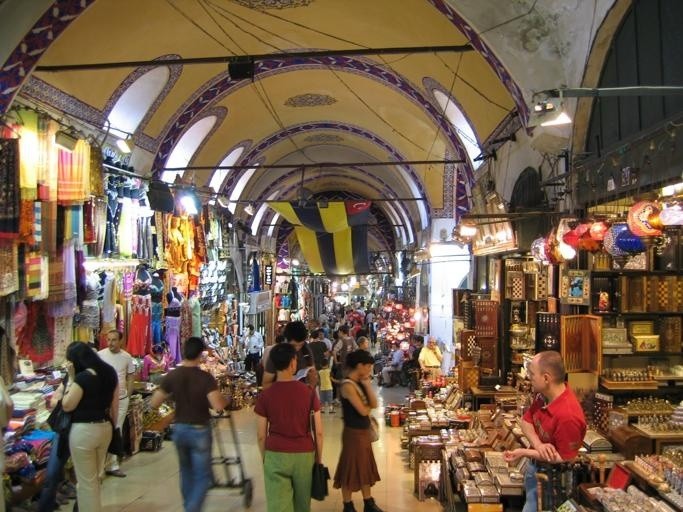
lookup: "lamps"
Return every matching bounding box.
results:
[55,125,81,152]
[527,86,683,127]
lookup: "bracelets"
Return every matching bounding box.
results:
[65,382,71,387]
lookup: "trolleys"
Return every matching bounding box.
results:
[209,402,252,507]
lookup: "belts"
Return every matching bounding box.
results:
[74,418,109,424]
[178,421,199,425]
[425,365,440,368]
[529,458,539,466]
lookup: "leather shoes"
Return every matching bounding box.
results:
[106,469,126,477]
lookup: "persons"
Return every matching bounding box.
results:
[331,349,385,512]
[61,340,120,511]
[95,330,136,478]
[501,350,587,512]
[252,342,323,512]
[33,371,77,511]
[149,273,164,354]
[82,274,101,332]
[125,263,155,356]
[150,336,230,511]
[262,296,444,414]
[164,286,183,363]
[0,374,14,512]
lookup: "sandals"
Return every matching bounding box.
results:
[58,482,76,498]
[56,492,69,504]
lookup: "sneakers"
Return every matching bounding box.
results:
[344,502,356,512]
[363,499,383,512]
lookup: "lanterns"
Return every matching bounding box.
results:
[528,199,682,266]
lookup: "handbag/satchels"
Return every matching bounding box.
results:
[368,415,380,442]
[46,391,72,433]
[312,463,330,501]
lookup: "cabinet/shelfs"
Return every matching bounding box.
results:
[592,269,683,402]
